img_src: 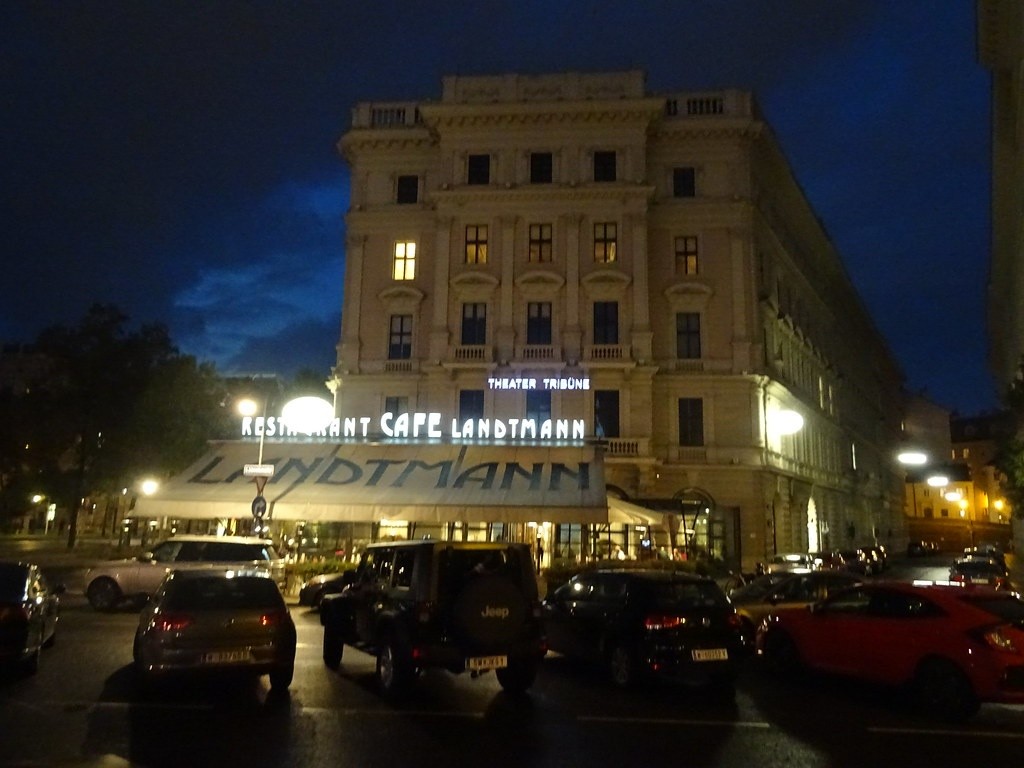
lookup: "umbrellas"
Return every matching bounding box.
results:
[593,495,664,564]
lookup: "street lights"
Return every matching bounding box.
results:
[236,391,336,534]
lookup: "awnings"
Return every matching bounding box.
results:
[133,444,608,518]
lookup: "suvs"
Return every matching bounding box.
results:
[81,532,289,611]
[319,539,550,697]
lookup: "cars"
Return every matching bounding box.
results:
[131,566,298,692]
[299,573,355,608]
[0,560,66,679]
[540,568,756,697]
[759,578,1024,716]
[756,540,1010,592]
